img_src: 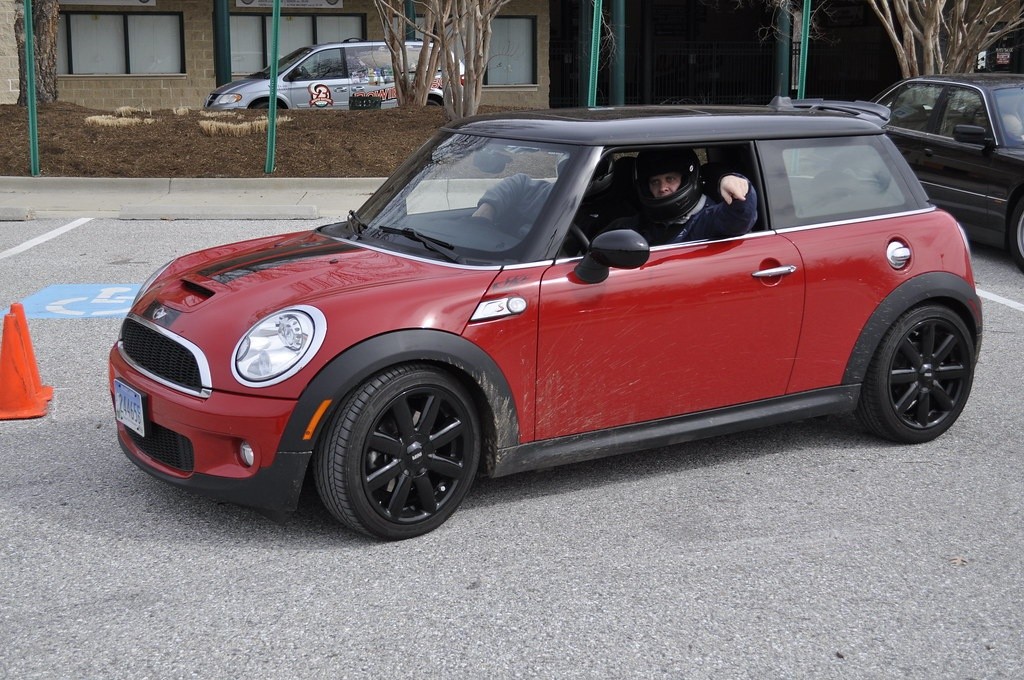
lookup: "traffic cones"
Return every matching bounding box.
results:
[11,303,53,402]
[0,314,47,420]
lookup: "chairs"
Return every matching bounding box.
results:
[794,169,888,221]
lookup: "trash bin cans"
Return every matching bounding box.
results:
[349,96,381,110]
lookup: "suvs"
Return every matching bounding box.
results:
[203,38,465,111]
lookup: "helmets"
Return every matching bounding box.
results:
[636,147,704,221]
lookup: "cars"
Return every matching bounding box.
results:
[868,73,1024,272]
[108,95,982,541]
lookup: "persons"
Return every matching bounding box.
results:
[472,150,615,239]
[589,146,758,246]
